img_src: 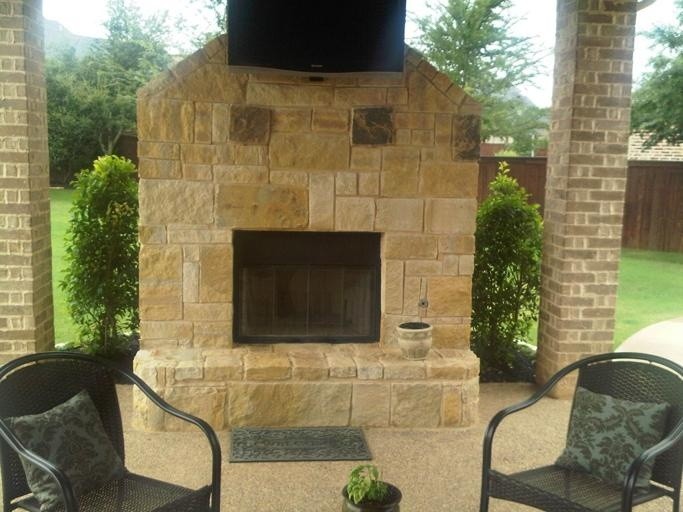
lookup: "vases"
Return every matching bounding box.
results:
[397,323,434,360]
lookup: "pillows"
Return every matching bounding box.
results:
[555,385,671,495]
[0,388,131,512]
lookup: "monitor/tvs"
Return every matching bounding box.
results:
[226,0,407,80]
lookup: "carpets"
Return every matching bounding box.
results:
[230,426,374,464]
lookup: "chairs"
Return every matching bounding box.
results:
[0,350,222,512]
[480,352,683,512]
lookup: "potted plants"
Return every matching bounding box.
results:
[342,464,402,512]
